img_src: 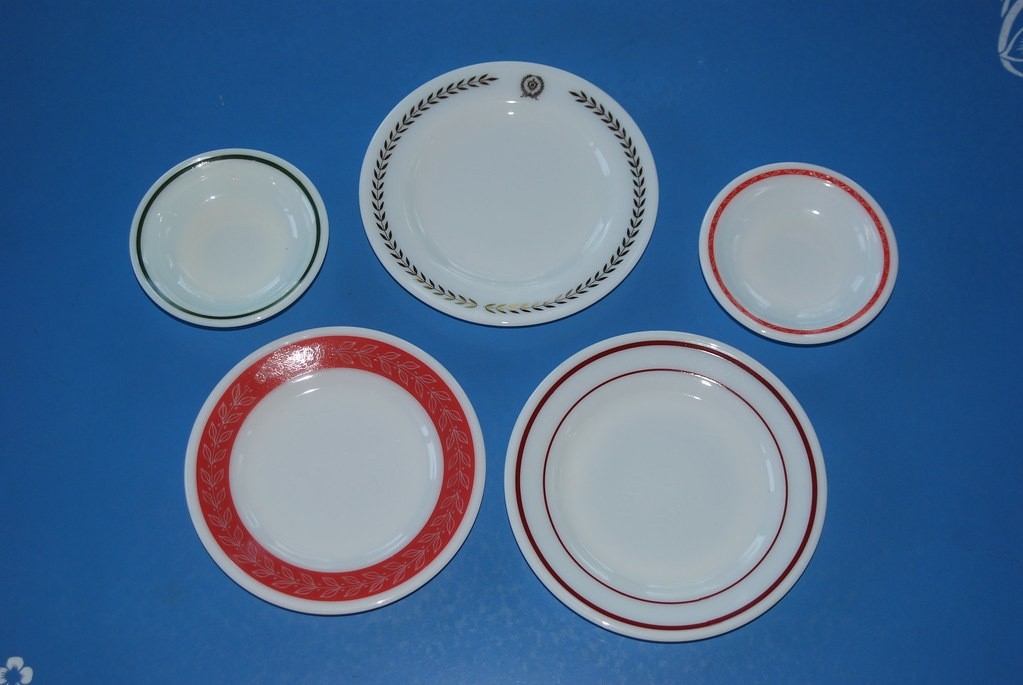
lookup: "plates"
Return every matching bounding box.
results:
[183,326,486,616]
[128,147,329,328]
[358,60,659,328]
[698,162,899,345]
[504,330,829,644]
